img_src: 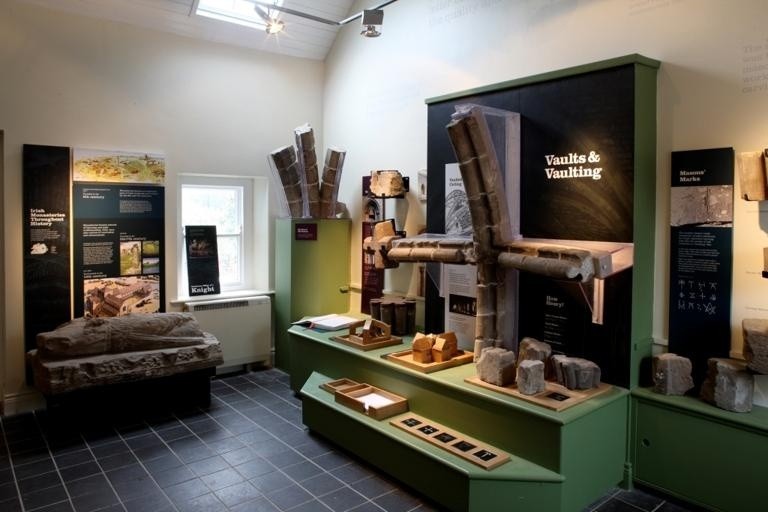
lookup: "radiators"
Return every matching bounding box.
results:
[184,296,272,373]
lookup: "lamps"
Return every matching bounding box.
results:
[244,0,385,38]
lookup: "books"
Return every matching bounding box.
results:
[290,313,358,331]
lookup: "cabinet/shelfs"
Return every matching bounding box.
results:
[276,217,351,371]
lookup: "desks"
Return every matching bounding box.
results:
[628,386,768,512]
[289,311,628,512]
[28,332,223,448]
